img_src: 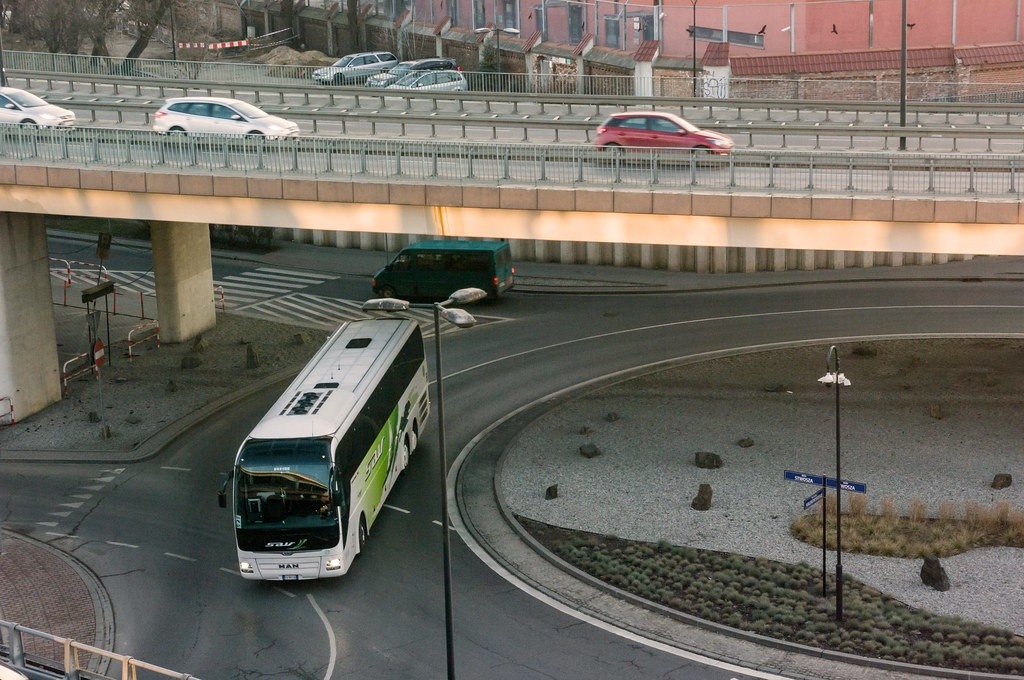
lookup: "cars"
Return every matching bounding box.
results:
[383,68,468,101]
[0,86,77,134]
[151,96,301,152]
[594,108,735,167]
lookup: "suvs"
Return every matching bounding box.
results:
[365,56,458,89]
[310,50,399,86]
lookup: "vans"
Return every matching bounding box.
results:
[370,238,515,307]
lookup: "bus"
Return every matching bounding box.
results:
[215,316,431,584]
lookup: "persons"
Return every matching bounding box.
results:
[320,490,330,512]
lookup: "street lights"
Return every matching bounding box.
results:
[360,286,490,680]
[817,344,852,621]
